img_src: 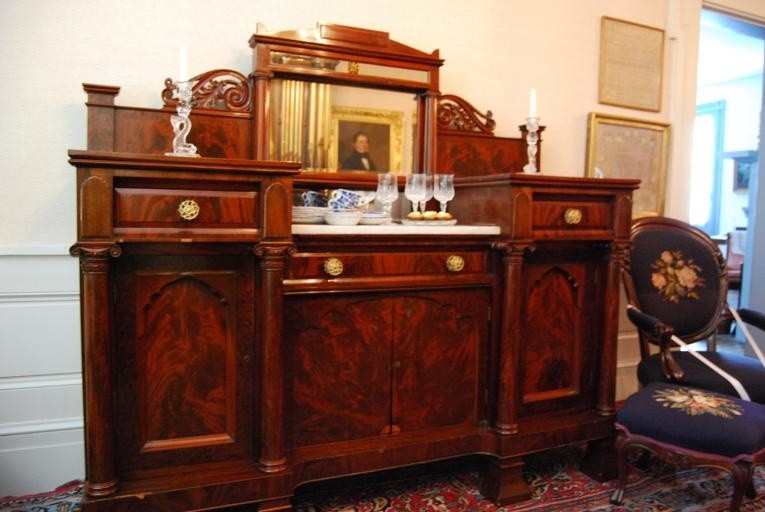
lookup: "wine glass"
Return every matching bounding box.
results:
[373,173,399,219]
[404,172,455,213]
[359,191,376,210]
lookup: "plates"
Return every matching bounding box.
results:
[400,217,458,226]
[290,206,388,226]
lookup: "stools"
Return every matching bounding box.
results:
[612,382,765,511]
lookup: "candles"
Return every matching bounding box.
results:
[530,87,537,116]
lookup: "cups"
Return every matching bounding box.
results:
[300,188,364,212]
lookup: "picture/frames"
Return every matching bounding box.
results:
[584,112,671,222]
[732,157,756,194]
[323,106,404,175]
[598,16,665,114]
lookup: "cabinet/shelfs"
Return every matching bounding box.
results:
[68,25,640,512]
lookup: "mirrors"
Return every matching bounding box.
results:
[247,24,443,191]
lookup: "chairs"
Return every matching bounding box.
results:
[621,216,765,501]
[724,227,746,286]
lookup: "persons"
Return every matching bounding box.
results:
[340,131,375,170]
